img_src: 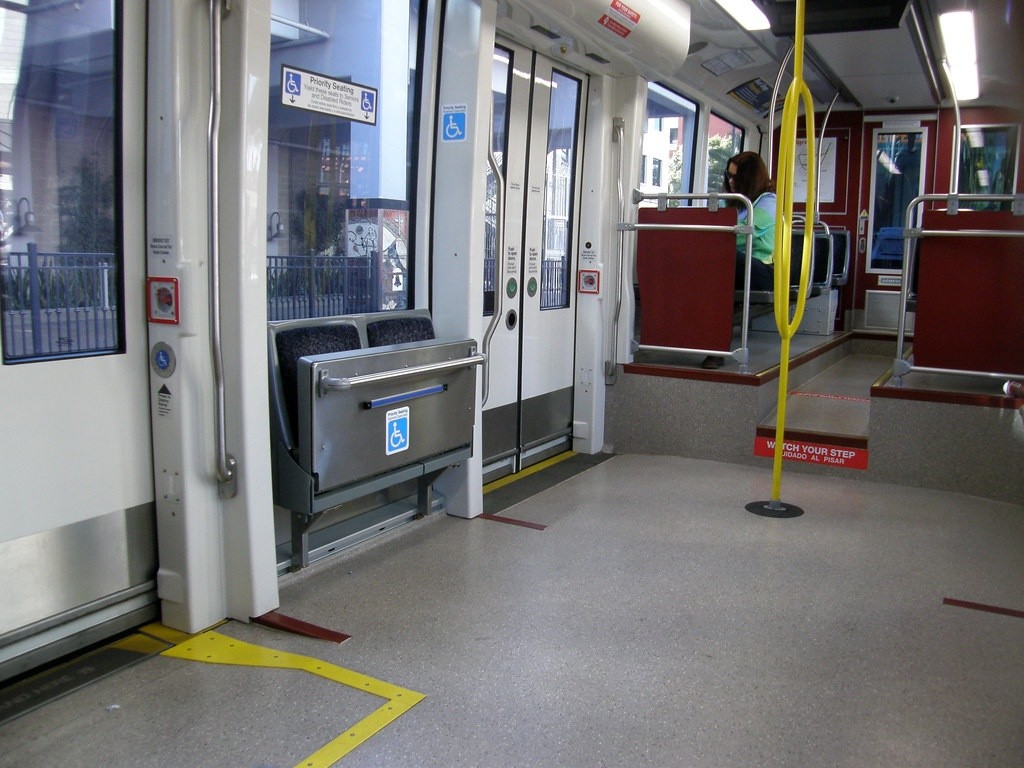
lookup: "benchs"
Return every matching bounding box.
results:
[265,307,492,573]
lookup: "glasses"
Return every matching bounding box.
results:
[725,170,737,180]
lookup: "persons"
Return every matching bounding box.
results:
[698,151,776,370]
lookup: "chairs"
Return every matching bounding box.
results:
[732,213,852,324]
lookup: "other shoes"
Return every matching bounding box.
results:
[702,356,724,368]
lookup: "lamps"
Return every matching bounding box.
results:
[14,196,45,233]
[268,212,289,242]
